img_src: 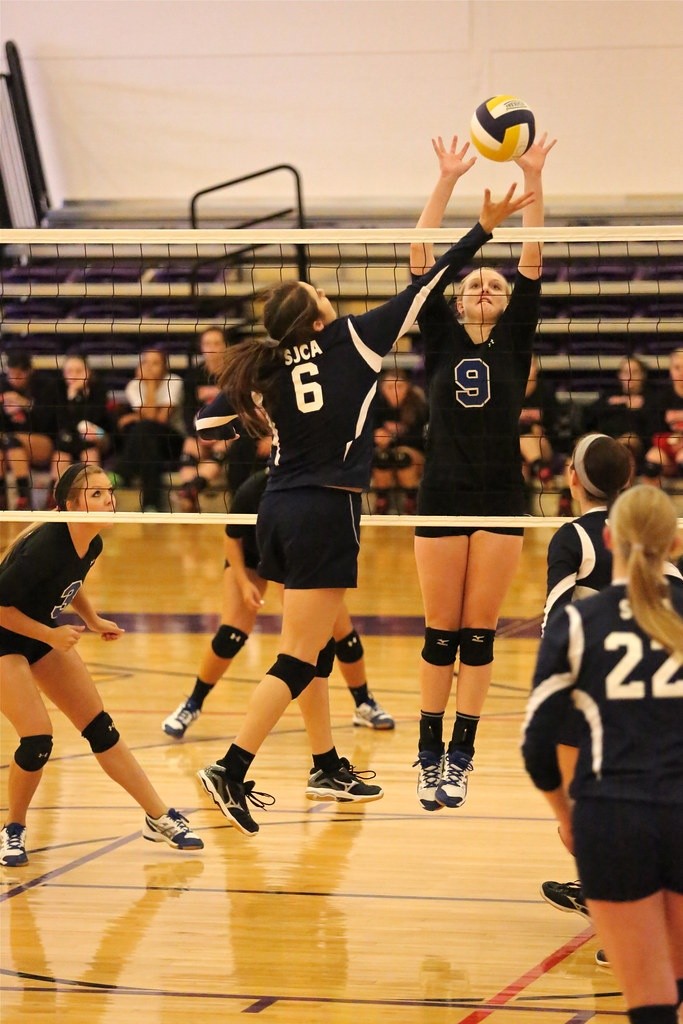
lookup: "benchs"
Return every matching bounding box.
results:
[0,194,683,403]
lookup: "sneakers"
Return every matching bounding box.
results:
[412,742,445,811]
[594,949,611,967]
[353,691,394,729]
[304,757,384,803]
[541,880,593,924]
[0,823,29,866]
[197,761,275,837]
[142,808,204,850]
[162,693,206,739]
[435,741,475,808]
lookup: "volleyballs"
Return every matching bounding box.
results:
[467,91,538,165]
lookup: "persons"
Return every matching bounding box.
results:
[4,861,205,1023]
[195,181,537,837]
[0,465,203,869]
[163,437,394,739]
[415,957,479,1022]
[2,348,683,517]
[521,432,683,1023]
[164,728,378,990]
[408,134,559,812]
